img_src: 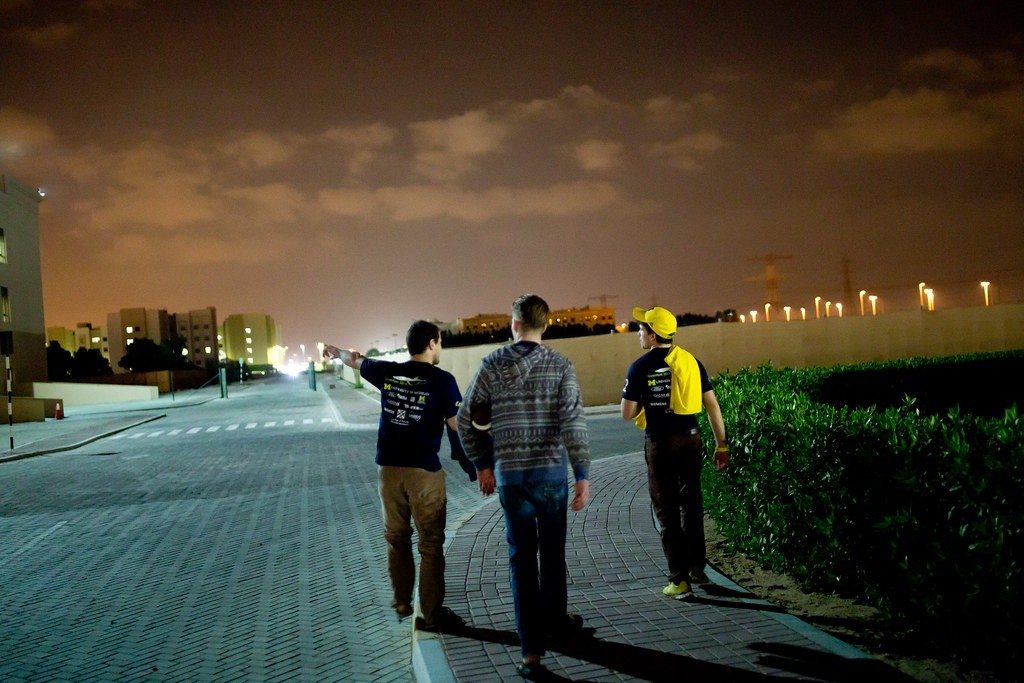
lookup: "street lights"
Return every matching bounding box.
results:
[824,300,831,318]
[868,294,878,316]
[749,310,758,322]
[859,289,867,316]
[918,281,926,310]
[800,307,806,320]
[835,302,843,317]
[764,303,771,322]
[980,281,990,306]
[924,289,935,311]
[815,296,822,318]
[783,305,792,321]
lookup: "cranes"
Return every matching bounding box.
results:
[585,291,619,307]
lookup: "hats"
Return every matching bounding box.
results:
[633,306,677,339]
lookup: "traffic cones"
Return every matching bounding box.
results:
[53,400,64,420]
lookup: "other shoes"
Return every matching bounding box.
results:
[427,607,449,627]
[559,615,583,632]
[517,661,540,676]
[396,606,414,617]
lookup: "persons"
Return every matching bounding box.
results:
[321,319,476,626]
[621,307,730,601]
[456,290,593,681]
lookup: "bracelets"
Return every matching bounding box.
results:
[714,446,728,453]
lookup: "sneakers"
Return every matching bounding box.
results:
[662,580,692,599]
[691,573,709,584]
